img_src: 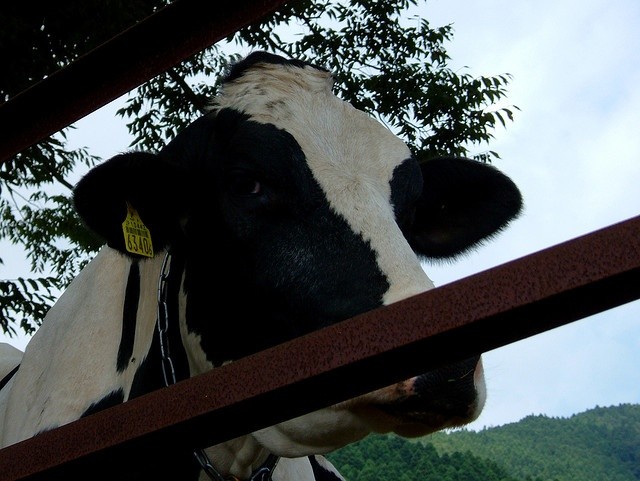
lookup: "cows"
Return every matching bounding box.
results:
[0,50,522,481]
